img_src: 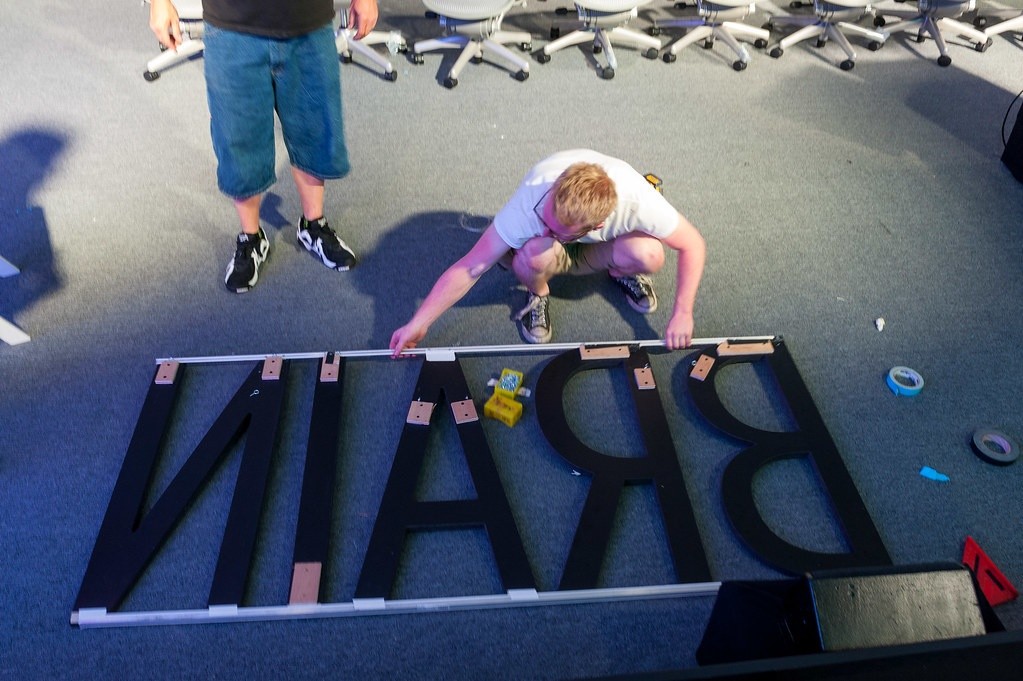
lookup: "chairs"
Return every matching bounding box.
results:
[143,0,205,82]
[537,0,662,80]
[761,0,885,71]
[648,0,769,72]
[410,0,533,89]
[873,0,993,53]
[333,0,408,82]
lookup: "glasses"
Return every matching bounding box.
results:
[532,186,597,244]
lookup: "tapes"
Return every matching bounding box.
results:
[972,426,1020,464]
[885,365,924,398]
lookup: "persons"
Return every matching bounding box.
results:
[150,0,378,293]
[389,148,707,359]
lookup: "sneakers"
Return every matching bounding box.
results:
[296,212,358,272]
[616,273,657,314]
[514,288,553,344]
[224,226,271,294]
